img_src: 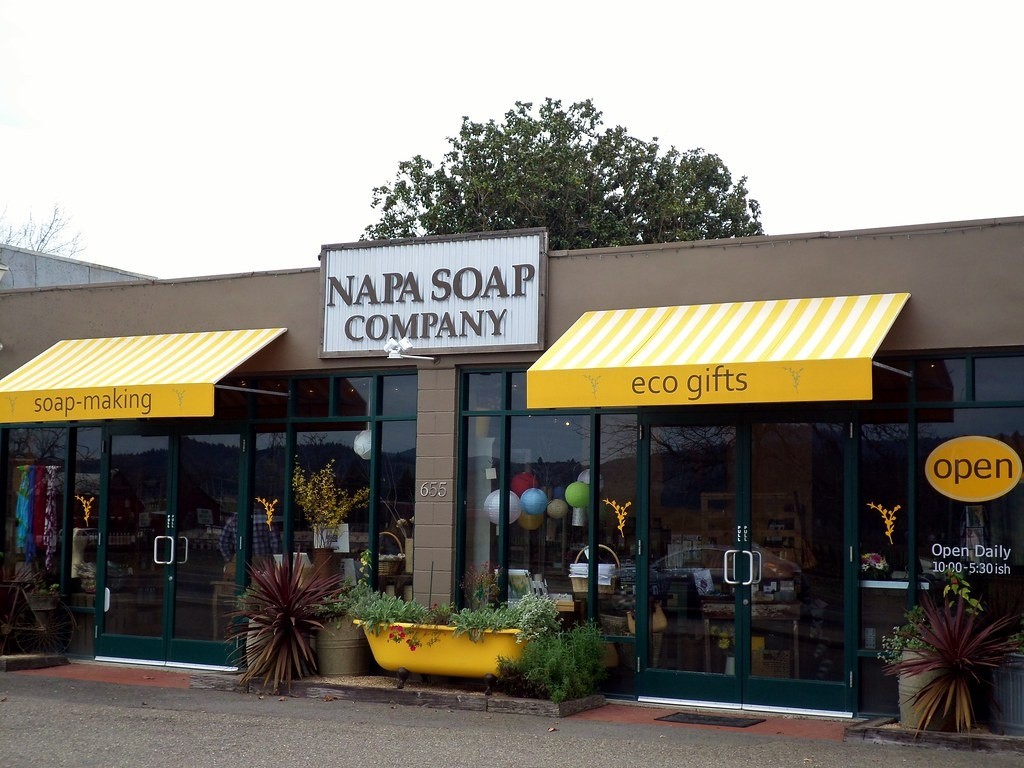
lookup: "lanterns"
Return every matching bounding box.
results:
[484,469,604,537]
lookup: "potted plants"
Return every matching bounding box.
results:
[291,452,368,578]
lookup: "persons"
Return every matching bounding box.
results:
[218,486,281,562]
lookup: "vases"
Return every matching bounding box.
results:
[897,645,953,731]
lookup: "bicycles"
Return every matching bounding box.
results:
[0,577,78,659]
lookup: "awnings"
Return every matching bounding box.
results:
[526,292,913,409]
[0,328,288,422]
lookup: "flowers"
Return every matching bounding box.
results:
[878,603,938,665]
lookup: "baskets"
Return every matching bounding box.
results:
[568,544,621,594]
[599,607,628,633]
[622,632,663,668]
[751,648,793,680]
[378,532,405,576]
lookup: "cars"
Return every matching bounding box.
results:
[606,543,802,606]
